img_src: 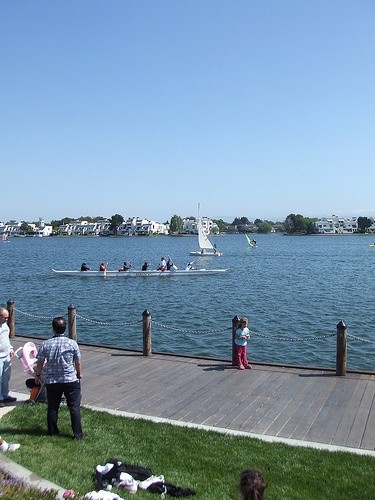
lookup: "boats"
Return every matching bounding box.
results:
[369,244,375,246]
[240,230,258,249]
[52,268,231,277]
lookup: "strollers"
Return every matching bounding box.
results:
[15,342,67,406]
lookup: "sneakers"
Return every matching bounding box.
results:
[245,365,252,369]
[6,443,20,451]
[122,483,138,494]
[138,474,165,489]
[238,365,245,369]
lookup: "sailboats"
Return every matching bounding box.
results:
[187,202,225,257]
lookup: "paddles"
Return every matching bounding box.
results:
[104,262,108,278]
[168,255,178,270]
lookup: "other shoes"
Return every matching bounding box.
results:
[4,396,16,402]
[0,401,5,406]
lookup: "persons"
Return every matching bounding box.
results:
[124,262,131,271]
[100,263,107,271]
[81,262,91,272]
[251,239,257,245]
[35,317,86,440]
[213,244,217,254]
[0,307,17,407]
[235,317,252,370]
[239,469,267,500]
[142,262,149,271]
[166,259,174,271]
[0,435,22,452]
[156,257,167,271]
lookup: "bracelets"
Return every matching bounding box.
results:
[36,373,40,377]
